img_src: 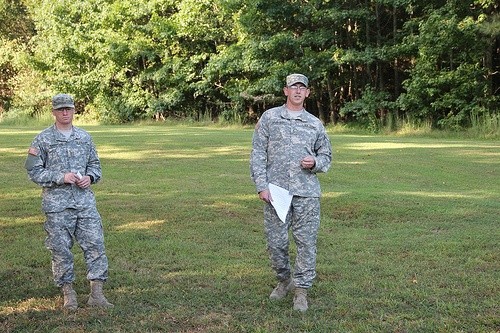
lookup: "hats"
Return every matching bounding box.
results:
[286,73,308,88]
[51,94,74,110]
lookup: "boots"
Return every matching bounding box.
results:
[270,278,293,299]
[60,283,78,309]
[87,280,114,308]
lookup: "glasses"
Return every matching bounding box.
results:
[288,85,308,90]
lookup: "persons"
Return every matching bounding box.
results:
[250,74,332,314]
[24,94,114,309]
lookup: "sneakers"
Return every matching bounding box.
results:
[293,287,308,311]
